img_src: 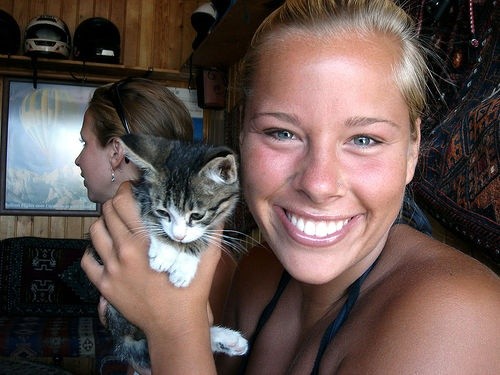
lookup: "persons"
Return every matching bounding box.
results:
[80,0,500,375]
[74,79,239,327]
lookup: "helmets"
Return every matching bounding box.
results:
[190,2,217,20]
[0,11,21,55]
[23,14,71,57]
[73,16,121,65]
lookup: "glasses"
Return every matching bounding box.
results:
[110,80,131,134]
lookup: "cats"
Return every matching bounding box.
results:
[82,133,266,375]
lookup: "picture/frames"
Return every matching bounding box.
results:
[0,75,103,219]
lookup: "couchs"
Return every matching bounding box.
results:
[0,236,127,375]
